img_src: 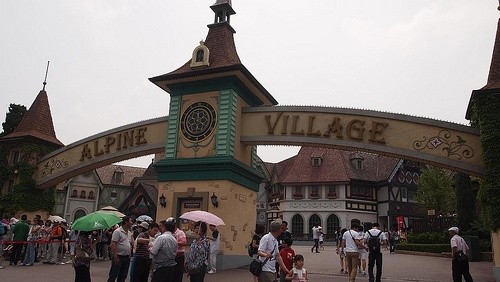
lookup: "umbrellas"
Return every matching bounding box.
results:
[71,211,123,231]
[179,211,226,234]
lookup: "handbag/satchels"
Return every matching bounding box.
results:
[249,258,263,277]
[83,252,96,261]
[248,242,255,257]
[453,250,465,261]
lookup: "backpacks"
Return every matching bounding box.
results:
[367,230,382,255]
[52,226,67,240]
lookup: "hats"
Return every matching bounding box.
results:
[166,217,176,223]
[448,227,459,233]
[9,218,20,225]
[53,218,60,223]
[140,221,149,229]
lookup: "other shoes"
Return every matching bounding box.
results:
[339,268,344,272]
[0,250,74,269]
[362,272,366,275]
[358,269,361,273]
[311,248,313,252]
[207,268,217,273]
[336,251,339,254]
[97,256,112,261]
[277,274,280,278]
[345,271,348,274]
[316,251,320,253]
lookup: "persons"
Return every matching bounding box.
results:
[107,216,220,282]
[72,230,100,282]
[251,218,308,282]
[336,223,388,282]
[311,224,325,253]
[448,227,473,282]
[0,214,80,268]
[93,224,119,261]
[384,227,407,253]
